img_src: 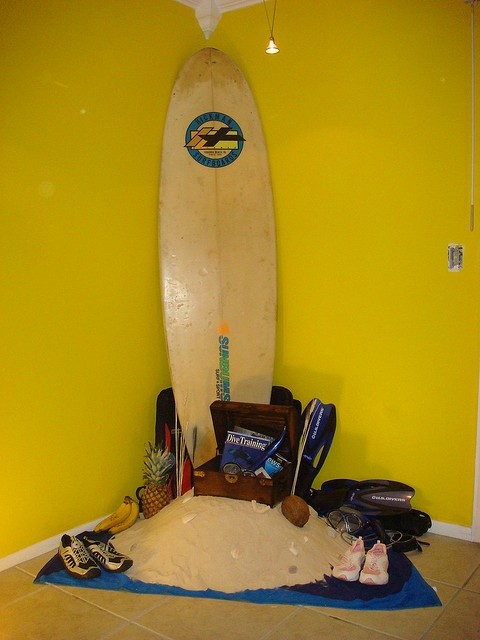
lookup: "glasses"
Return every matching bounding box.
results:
[327,509,362,533]
[222,462,256,478]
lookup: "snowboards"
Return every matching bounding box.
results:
[157,47,279,467]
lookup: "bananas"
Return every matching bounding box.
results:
[93,495,140,535]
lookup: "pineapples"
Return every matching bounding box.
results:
[142,442,175,519]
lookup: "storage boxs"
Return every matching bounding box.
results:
[193,399,297,508]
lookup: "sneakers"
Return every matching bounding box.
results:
[82,531,134,575]
[331,535,365,582]
[360,540,390,586]
[57,533,100,580]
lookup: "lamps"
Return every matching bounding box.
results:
[263,0,282,53]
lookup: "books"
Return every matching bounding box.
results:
[218,430,270,473]
